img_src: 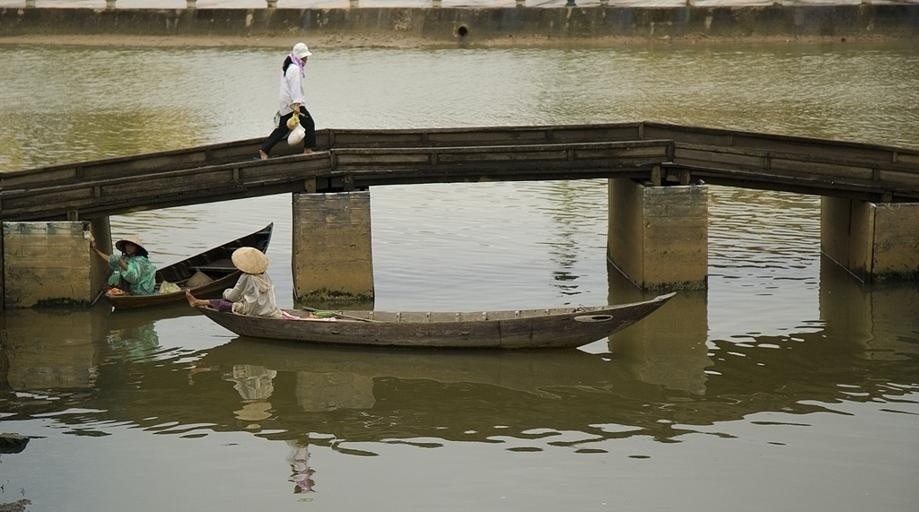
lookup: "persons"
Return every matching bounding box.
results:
[92,236,156,295]
[259,43,315,159]
[186,246,282,319]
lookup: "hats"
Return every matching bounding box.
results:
[186,271,213,287]
[115,235,148,255]
[293,43,312,59]
[231,246,268,274]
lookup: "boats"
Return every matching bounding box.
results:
[106,224,272,311]
[197,292,681,349]
[197,340,664,410]
[109,297,200,329]
